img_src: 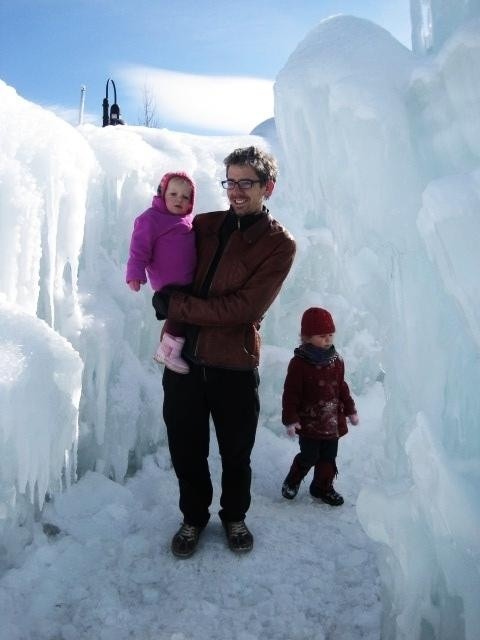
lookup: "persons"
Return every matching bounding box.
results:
[127,170,197,375]
[281,307,359,506]
[152,146,297,558]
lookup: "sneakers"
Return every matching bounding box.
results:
[221,517,254,553]
[169,510,211,558]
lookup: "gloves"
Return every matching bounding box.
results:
[127,280,146,292]
[152,288,171,321]
[349,412,360,426]
[285,423,304,440]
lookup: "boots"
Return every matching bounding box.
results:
[281,457,308,500]
[310,461,344,507]
[152,330,190,376]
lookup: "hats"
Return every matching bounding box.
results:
[300,307,334,338]
[156,171,195,215]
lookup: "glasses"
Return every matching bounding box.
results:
[220,178,261,189]
